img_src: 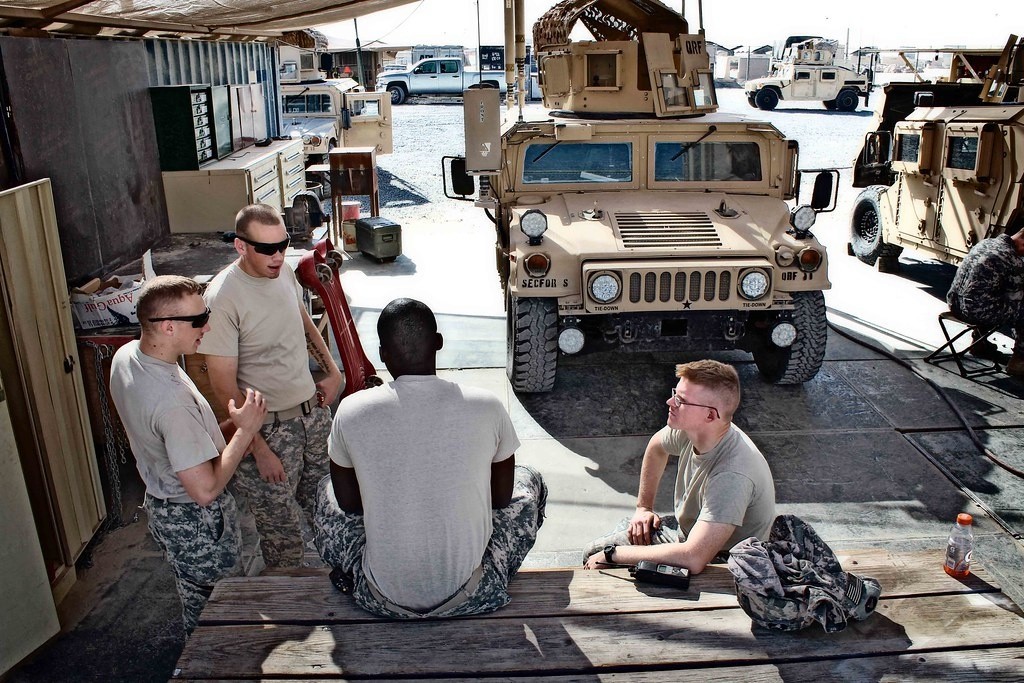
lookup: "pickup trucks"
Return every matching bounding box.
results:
[375,57,507,105]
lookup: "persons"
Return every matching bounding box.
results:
[583,359,776,575]
[314,298,547,621]
[197,202,343,568]
[945,227,1024,376]
[109,273,268,645]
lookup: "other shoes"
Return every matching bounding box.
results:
[1005,352,1024,376]
[970,339,998,353]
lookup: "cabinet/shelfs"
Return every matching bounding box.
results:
[163,141,381,245]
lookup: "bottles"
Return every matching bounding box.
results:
[943,513,974,578]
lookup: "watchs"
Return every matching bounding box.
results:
[603,544,616,563]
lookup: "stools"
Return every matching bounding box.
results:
[924,312,1002,379]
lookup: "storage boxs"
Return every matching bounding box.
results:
[355,216,402,263]
[69,248,156,329]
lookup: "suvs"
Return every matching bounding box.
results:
[441,0,841,396]
[850,34,1024,272]
[279,30,396,156]
[742,41,877,110]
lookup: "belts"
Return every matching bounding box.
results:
[263,394,318,425]
[365,563,483,617]
[169,495,196,503]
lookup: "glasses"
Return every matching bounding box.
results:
[672,387,721,419]
[234,232,291,256]
[148,305,212,328]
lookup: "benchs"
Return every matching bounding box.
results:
[167,536,1022,683]
